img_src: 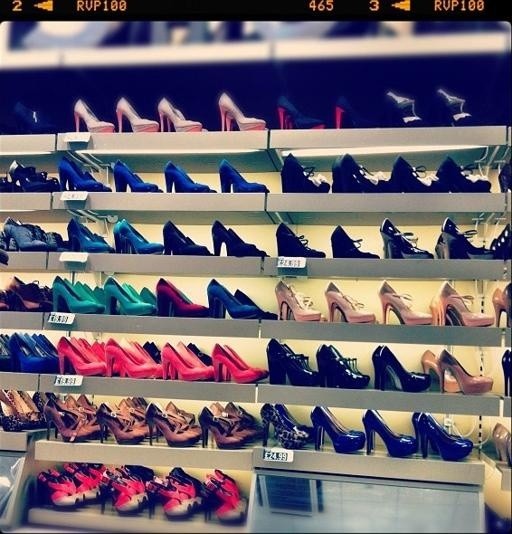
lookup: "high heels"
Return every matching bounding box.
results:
[218,92,269,132]
[36,462,248,523]
[385,90,426,127]
[116,97,159,133]
[276,95,326,130]
[0,151,512,193]
[13,102,57,135]
[73,99,115,133]
[434,88,473,127]
[0,216,512,260]
[157,97,208,132]
[0,273,512,328]
[0,389,510,464]
[334,95,380,129]
[0,332,512,396]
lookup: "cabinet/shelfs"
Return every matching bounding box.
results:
[0,127,511,533]
